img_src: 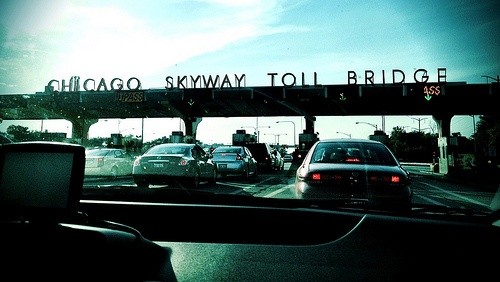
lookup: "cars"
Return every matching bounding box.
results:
[84,147,137,181]
[294,136,415,210]
[272,150,285,172]
[130,142,217,190]
[283,154,293,163]
[210,145,258,181]
[203,147,212,158]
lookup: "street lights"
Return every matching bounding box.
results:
[264,133,287,143]
[276,120,296,145]
[241,125,270,133]
[412,117,429,133]
[355,121,378,131]
[336,131,352,139]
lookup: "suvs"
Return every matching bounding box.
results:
[244,142,278,174]
